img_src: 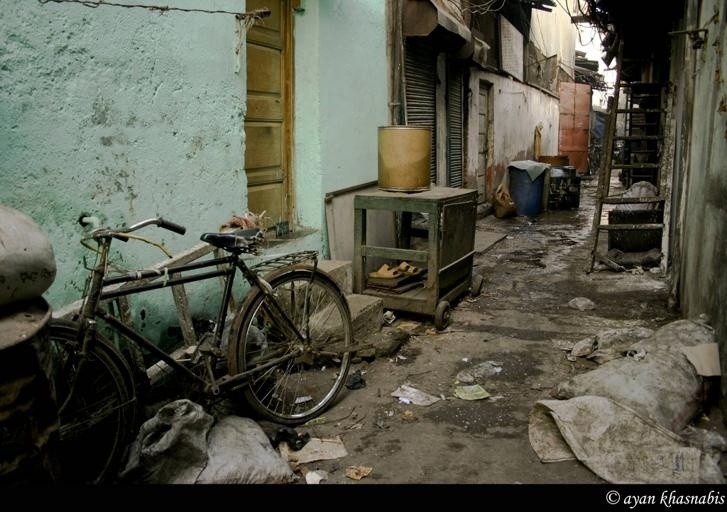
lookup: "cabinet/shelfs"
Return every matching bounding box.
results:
[352,184,478,315]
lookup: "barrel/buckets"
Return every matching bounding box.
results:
[538,154,569,167]
[377,125,432,191]
[508,166,544,213]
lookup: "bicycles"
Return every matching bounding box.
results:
[7,214,374,485]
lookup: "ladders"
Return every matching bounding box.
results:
[51,226,241,389]
[622,85,668,191]
[585,31,677,279]
